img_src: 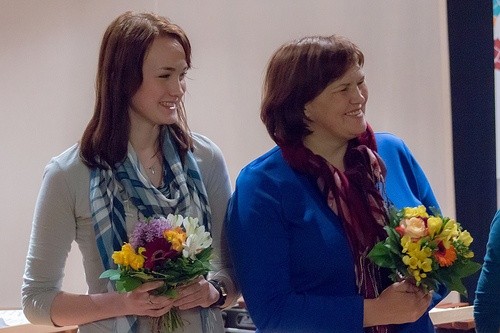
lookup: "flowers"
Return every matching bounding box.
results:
[99,213,217,333]
[367,205,483,299]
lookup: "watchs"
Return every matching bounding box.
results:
[208,280,227,307]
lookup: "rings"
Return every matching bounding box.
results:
[148,295,154,305]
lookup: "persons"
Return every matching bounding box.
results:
[20,12,241,333]
[470,208,500,333]
[221,35,453,332]
